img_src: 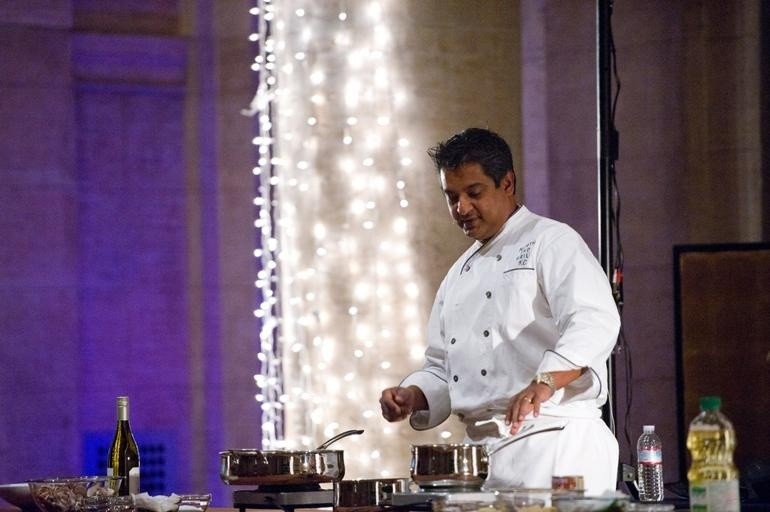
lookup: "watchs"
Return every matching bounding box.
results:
[533,371,556,395]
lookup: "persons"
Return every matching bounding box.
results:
[381,128,622,493]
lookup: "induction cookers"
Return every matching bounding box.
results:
[231,485,337,512]
[390,489,552,508]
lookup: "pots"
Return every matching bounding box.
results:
[218,429,364,486]
[337,477,415,507]
[410,426,565,491]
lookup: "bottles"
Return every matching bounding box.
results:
[107,396,140,496]
[686,395,740,512]
[637,425,664,502]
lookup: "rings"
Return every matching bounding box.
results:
[524,396,533,403]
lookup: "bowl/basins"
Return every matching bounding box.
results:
[429,497,631,512]
[169,494,212,512]
[0,476,136,512]
